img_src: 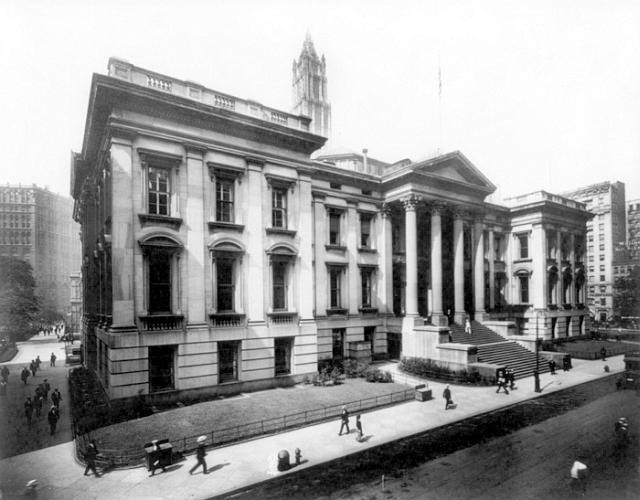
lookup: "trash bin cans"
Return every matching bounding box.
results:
[415,384,431,402]
[144,438,173,471]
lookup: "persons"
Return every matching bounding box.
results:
[337,405,349,435]
[562,355,572,372]
[548,358,556,375]
[442,383,451,410]
[1,350,63,435]
[148,444,167,477]
[354,413,363,442]
[84,438,101,478]
[188,439,209,475]
[495,368,517,395]
[600,346,606,361]
[464,316,472,335]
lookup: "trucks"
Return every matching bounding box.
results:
[616,350,640,391]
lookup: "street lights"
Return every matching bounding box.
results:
[533,310,550,394]
